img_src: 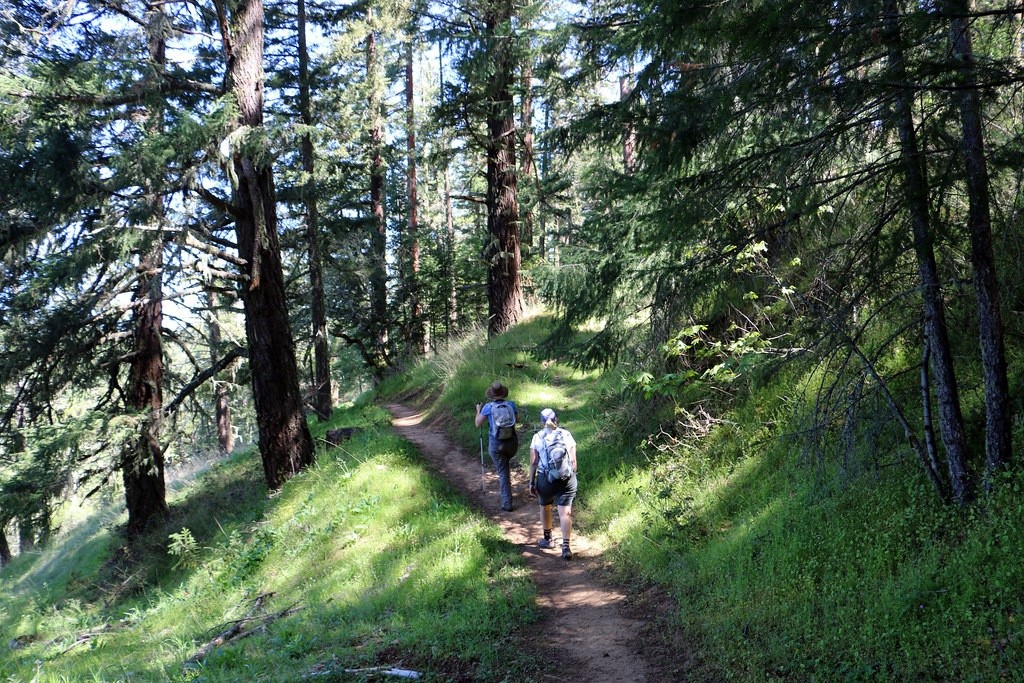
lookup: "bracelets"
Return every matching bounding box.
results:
[529,482,534,486]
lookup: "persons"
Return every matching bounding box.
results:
[475,381,519,513]
[528,407,577,559]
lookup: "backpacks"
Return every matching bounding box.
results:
[490,401,516,440]
[539,429,573,482]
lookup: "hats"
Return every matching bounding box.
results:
[540,408,556,422]
[485,382,509,400]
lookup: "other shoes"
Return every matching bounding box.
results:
[503,505,513,512]
[561,547,572,559]
[539,538,554,548]
